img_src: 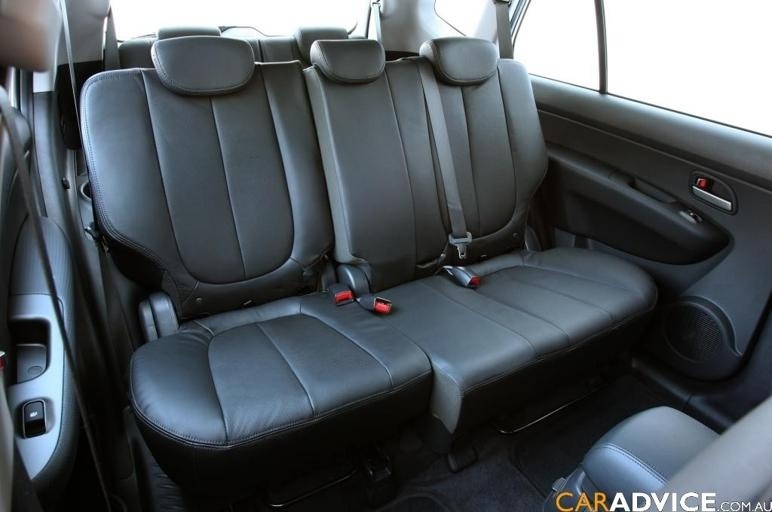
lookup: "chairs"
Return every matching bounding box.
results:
[69,17,772,512]
